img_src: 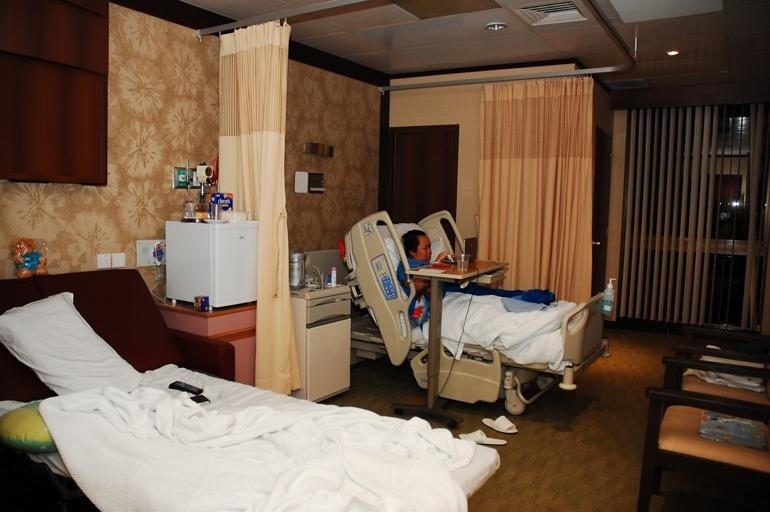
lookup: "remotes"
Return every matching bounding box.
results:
[169,381,202,394]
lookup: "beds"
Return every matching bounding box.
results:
[345,209,610,416]
[2,269,500,512]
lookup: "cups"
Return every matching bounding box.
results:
[211,204,221,220]
[456,253,470,271]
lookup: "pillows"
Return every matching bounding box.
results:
[2,292,140,395]
[385,238,449,297]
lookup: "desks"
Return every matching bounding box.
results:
[406,258,509,283]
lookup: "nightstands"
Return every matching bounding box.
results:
[160,303,256,390]
[291,284,351,403]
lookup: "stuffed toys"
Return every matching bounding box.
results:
[10,237,48,280]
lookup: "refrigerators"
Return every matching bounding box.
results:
[164,220,259,309]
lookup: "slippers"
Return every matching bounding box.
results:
[459,430,508,446]
[481,415,518,434]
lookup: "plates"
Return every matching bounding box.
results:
[203,218,228,224]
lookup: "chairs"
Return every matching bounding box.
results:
[646,356,770,406]
[660,323,770,403]
[639,386,770,511]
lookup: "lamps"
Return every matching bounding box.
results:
[483,21,507,32]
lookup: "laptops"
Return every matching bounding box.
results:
[443,238,477,263]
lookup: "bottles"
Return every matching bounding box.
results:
[331,266,336,287]
[199,183,205,204]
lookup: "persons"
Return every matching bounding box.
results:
[397,231,575,335]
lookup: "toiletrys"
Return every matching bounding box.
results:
[601,278,617,315]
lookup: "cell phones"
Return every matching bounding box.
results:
[190,395,210,405]
